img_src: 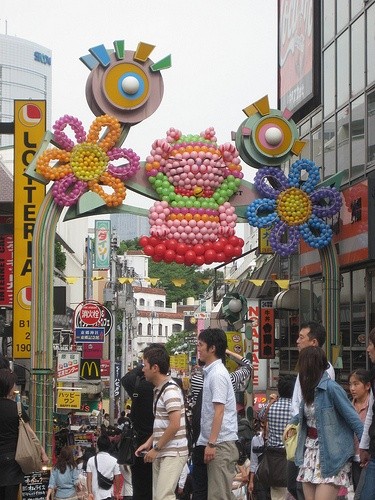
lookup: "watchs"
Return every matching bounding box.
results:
[207,442,216,448]
[154,444,161,451]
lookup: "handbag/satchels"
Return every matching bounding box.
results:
[95,454,114,490]
[285,434,297,461]
[256,447,287,487]
[253,400,275,435]
[15,402,42,475]
[117,437,133,465]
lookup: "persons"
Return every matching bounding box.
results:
[0,368,49,500]
[348,329,375,500]
[121,343,187,500]
[178,349,305,500]
[297,346,369,500]
[135,349,189,500]
[46,405,135,500]
[198,328,239,500]
[287,322,335,500]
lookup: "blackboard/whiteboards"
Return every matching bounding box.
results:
[20,468,51,500]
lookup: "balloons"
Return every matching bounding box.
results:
[36,114,141,207]
[139,126,245,266]
[247,159,344,257]
